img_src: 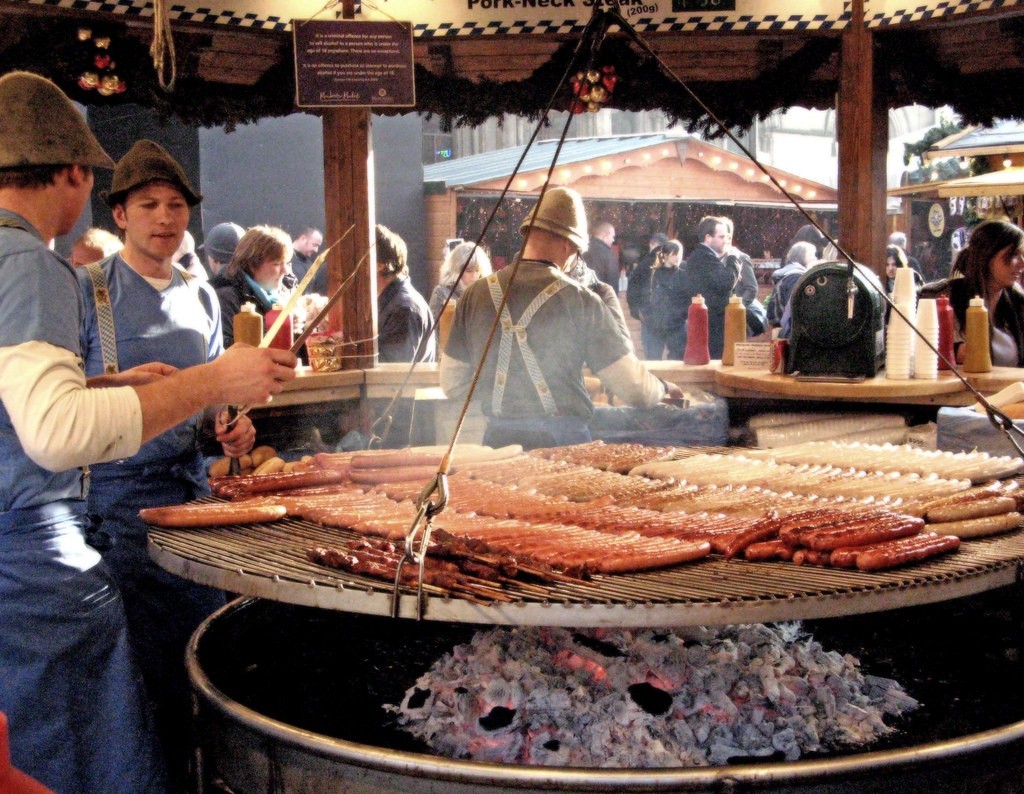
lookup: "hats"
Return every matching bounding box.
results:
[519,186,589,257]
[99,140,203,211]
[0,72,116,171]
[196,223,248,262]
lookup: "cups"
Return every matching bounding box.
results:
[885,268,938,379]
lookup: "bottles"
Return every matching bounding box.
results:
[724,243,751,261]
[937,293,956,370]
[722,294,746,365]
[617,265,629,293]
[438,297,457,361]
[684,293,710,365]
[963,295,992,372]
[232,301,262,347]
[263,301,293,351]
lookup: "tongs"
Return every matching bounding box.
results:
[216,222,377,429]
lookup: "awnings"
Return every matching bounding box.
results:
[938,166,1024,197]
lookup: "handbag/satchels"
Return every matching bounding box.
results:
[745,299,770,333]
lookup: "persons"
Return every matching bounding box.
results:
[768,224,837,326]
[585,220,619,297]
[441,188,685,448]
[0,70,298,794]
[376,224,435,363]
[685,217,768,361]
[570,254,636,350]
[80,140,255,794]
[627,234,687,360]
[918,220,1024,369]
[430,243,489,332]
[172,222,323,350]
[886,232,924,290]
[72,229,124,268]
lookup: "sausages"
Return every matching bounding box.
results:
[138,439,1024,574]
